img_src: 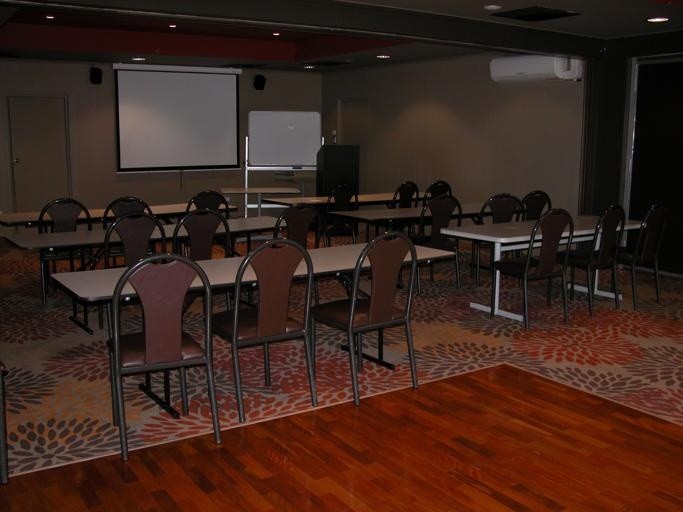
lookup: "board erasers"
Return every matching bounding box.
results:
[292,166,302,169]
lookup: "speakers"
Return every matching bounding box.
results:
[253,74,265,89]
[89,66,102,84]
[316,143,360,195]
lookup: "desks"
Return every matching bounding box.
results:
[439,213,648,321]
[330,205,491,244]
[263,193,445,209]
[2,202,244,229]
[0,214,303,335]
[49,240,458,420]
[220,185,302,213]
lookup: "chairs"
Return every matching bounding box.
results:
[106,254,222,462]
[171,209,232,262]
[422,177,453,206]
[417,196,462,281]
[520,190,551,222]
[477,193,522,224]
[210,240,319,423]
[104,215,166,270]
[102,194,155,227]
[386,181,419,210]
[490,207,574,329]
[318,184,359,242]
[610,201,666,310]
[38,198,94,295]
[556,203,627,315]
[273,205,319,251]
[184,190,232,216]
[308,231,425,408]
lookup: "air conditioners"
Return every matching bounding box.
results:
[488,55,581,82]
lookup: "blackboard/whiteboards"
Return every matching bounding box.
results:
[248,110,322,166]
